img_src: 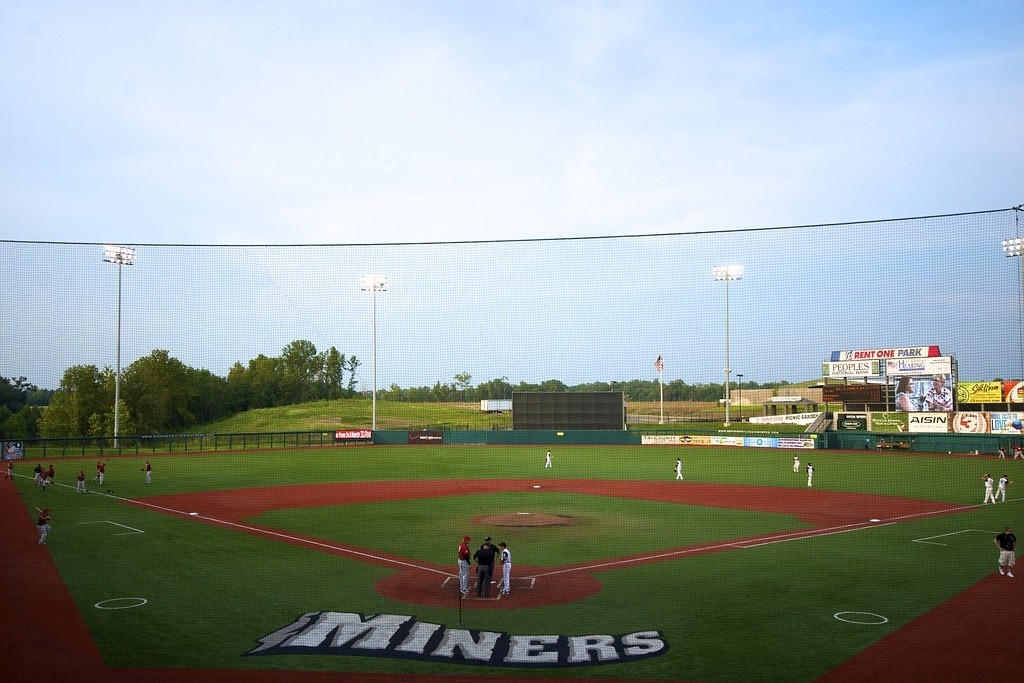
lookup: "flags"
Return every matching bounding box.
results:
[654,356,663,372]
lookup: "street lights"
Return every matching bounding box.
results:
[1001,237,1024,324]
[360,273,388,430]
[102,244,136,449]
[713,263,743,425]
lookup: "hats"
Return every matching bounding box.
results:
[484,536,491,541]
[498,542,506,547]
[484,543,490,546]
[464,535,472,541]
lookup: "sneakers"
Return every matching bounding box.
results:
[1006,572,1014,577]
[999,566,1005,575]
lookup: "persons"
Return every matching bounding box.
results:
[144,460,152,484]
[792,455,800,472]
[674,458,683,480]
[473,536,511,597]
[37,507,52,545]
[806,463,814,487]
[34,464,55,490]
[458,536,471,595]
[998,442,1024,460]
[994,475,1011,502]
[93,462,106,484]
[895,374,953,413]
[993,527,1018,577]
[4,460,13,480]
[981,473,996,504]
[76,470,87,494]
[545,450,552,468]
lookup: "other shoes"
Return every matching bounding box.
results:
[460,589,470,594]
[503,589,511,591]
[502,591,509,595]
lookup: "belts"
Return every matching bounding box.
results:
[508,561,509,563]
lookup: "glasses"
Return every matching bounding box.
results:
[1008,530,1012,532]
